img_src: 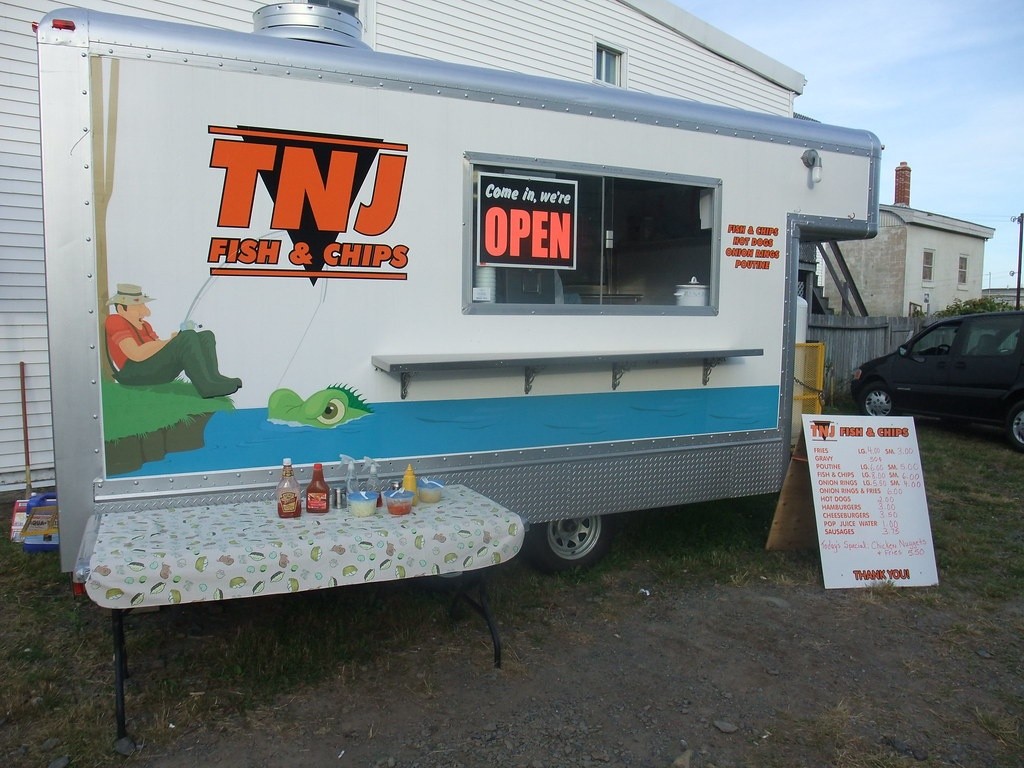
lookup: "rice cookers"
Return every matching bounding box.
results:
[674,276,710,307]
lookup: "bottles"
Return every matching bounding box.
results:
[277,458,302,518]
[306,464,330,513]
[403,464,417,506]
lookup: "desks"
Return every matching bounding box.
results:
[73,484,527,757]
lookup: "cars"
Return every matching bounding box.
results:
[850,311,1023,450]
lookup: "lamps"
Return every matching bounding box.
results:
[801,149,823,183]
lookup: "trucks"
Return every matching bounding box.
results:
[32,3,887,573]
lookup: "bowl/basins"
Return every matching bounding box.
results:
[418,479,445,502]
[384,490,414,515]
[347,492,379,517]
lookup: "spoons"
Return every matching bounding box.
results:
[421,476,444,488]
[390,487,405,498]
[360,491,369,500]
